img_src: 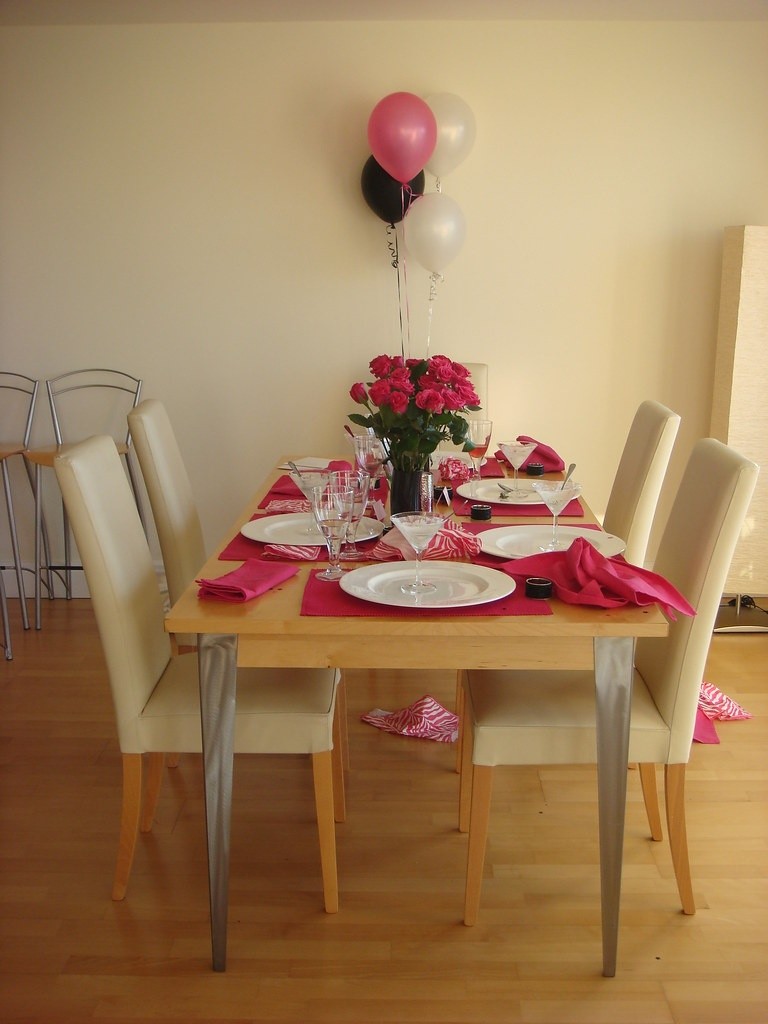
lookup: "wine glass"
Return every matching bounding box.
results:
[497,440,538,497]
[464,419,492,483]
[533,481,583,555]
[289,424,398,582]
[391,511,444,594]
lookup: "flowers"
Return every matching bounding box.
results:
[346,353,483,471]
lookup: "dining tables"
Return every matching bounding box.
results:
[164,354,699,979]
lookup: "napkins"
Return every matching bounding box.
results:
[493,435,566,472]
[271,459,353,496]
[194,558,302,604]
[498,537,698,622]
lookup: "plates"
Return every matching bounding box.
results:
[456,478,583,505]
[240,512,385,546]
[340,560,516,609]
[475,524,627,561]
[421,450,488,470]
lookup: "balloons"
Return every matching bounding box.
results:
[361,92,477,277]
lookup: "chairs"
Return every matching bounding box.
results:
[458,434,759,929]
[459,399,682,771]
[54,435,349,914]
[129,398,353,783]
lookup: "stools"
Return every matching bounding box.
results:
[0,369,59,633]
[25,367,143,632]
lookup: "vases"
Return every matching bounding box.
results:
[390,467,434,528]
